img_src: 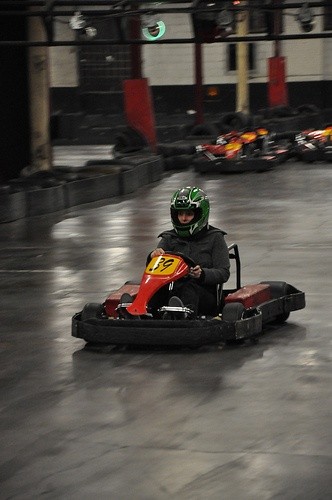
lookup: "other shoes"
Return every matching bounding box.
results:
[120,293,137,319]
[169,296,188,320]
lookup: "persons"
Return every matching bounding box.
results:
[120,186,230,319]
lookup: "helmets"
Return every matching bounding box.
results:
[170,186,209,240]
